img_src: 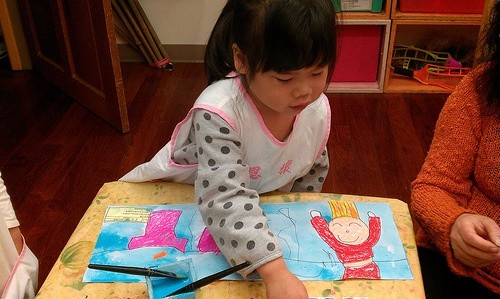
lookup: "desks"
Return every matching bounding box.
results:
[35,180,426,299]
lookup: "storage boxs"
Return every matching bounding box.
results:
[327,25,382,83]
[398,0,485,15]
[330,0,383,13]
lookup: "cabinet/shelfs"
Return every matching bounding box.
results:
[325,0,496,95]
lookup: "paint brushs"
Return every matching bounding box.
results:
[163,260,252,298]
[87,264,188,278]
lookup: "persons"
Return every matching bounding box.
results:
[0,175,40,299]
[118,0,340,299]
[411,0,500,299]
